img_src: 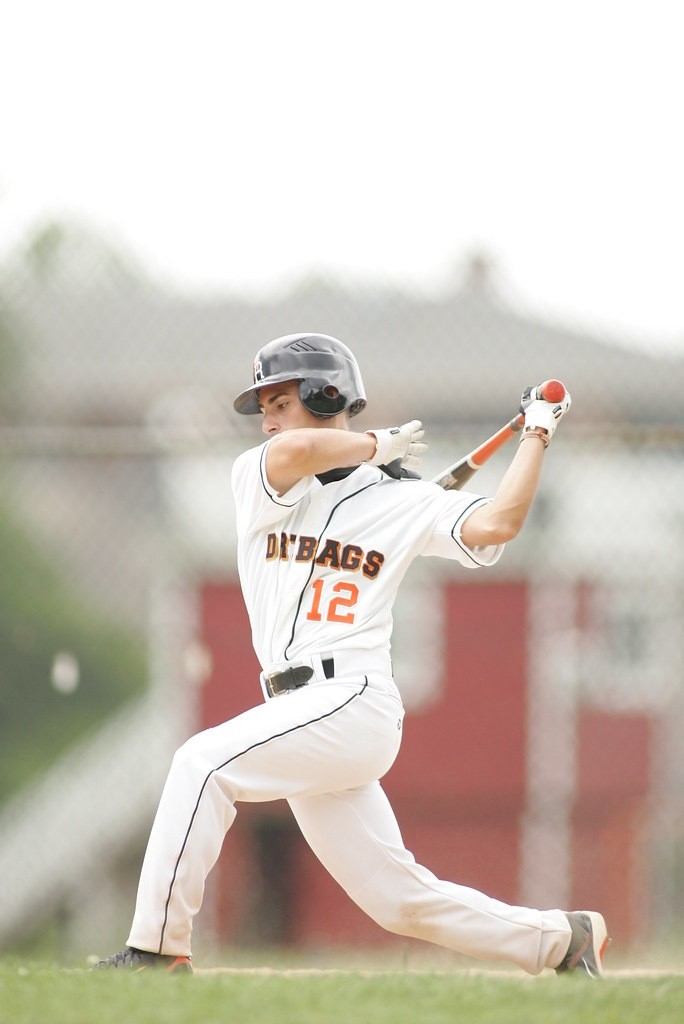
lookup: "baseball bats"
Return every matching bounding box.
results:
[432,381,566,490]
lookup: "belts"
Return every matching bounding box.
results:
[262,657,335,698]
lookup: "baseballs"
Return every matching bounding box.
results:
[51,653,79,691]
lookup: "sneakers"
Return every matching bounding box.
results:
[51,944,195,992]
[554,911,611,979]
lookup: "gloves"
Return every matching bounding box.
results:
[518,380,572,439]
[363,419,430,481]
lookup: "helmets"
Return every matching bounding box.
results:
[233,332,367,419]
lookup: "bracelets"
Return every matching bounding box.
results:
[519,431,551,448]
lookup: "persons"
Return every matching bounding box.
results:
[89,333,611,981]
